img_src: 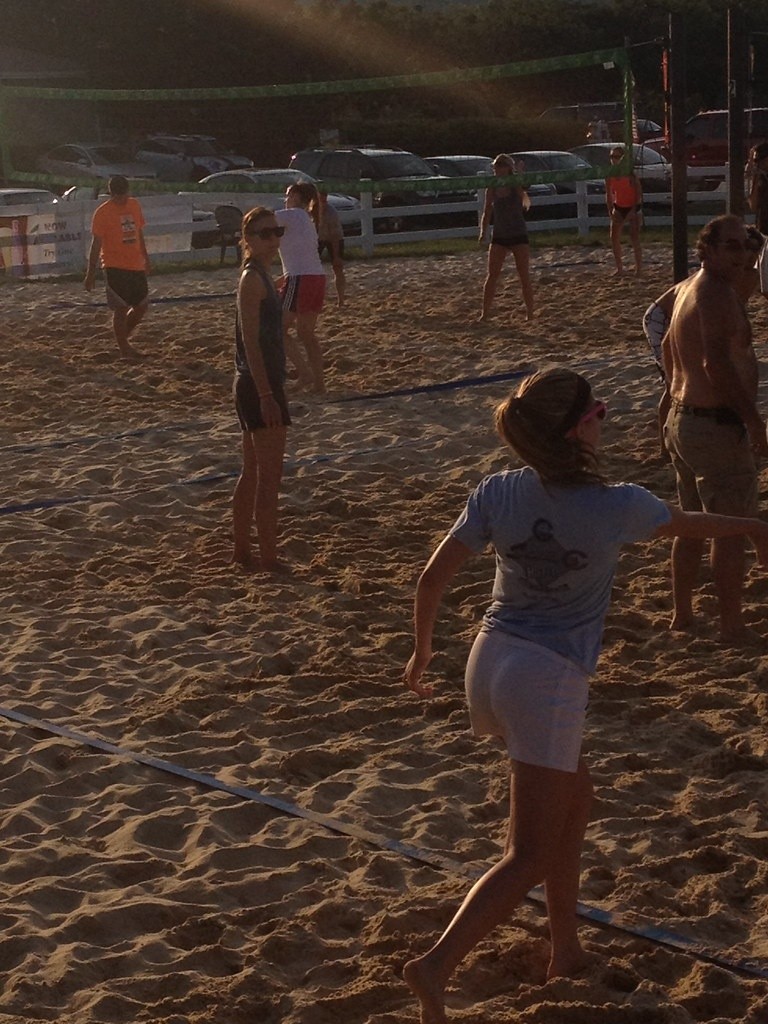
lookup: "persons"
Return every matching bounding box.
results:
[606,146,644,274]
[272,183,328,395]
[83,177,152,357]
[643,216,768,645]
[309,191,345,306]
[231,205,290,570]
[477,154,534,320]
[401,364,768,1023]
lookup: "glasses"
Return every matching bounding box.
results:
[754,157,766,162]
[609,154,621,159]
[747,244,762,252]
[492,162,510,168]
[248,226,286,240]
[566,400,607,441]
[713,239,751,252]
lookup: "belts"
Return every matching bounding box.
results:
[670,400,737,418]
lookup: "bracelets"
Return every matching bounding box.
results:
[259,391,274,399]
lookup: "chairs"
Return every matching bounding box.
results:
[216,205,246,265]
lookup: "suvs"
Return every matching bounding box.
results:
[539,101,768,191]
[288,145,475,229]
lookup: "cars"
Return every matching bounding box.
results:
[41,132,255,182]
[0,170,358,248]
[425,142,673,200]
